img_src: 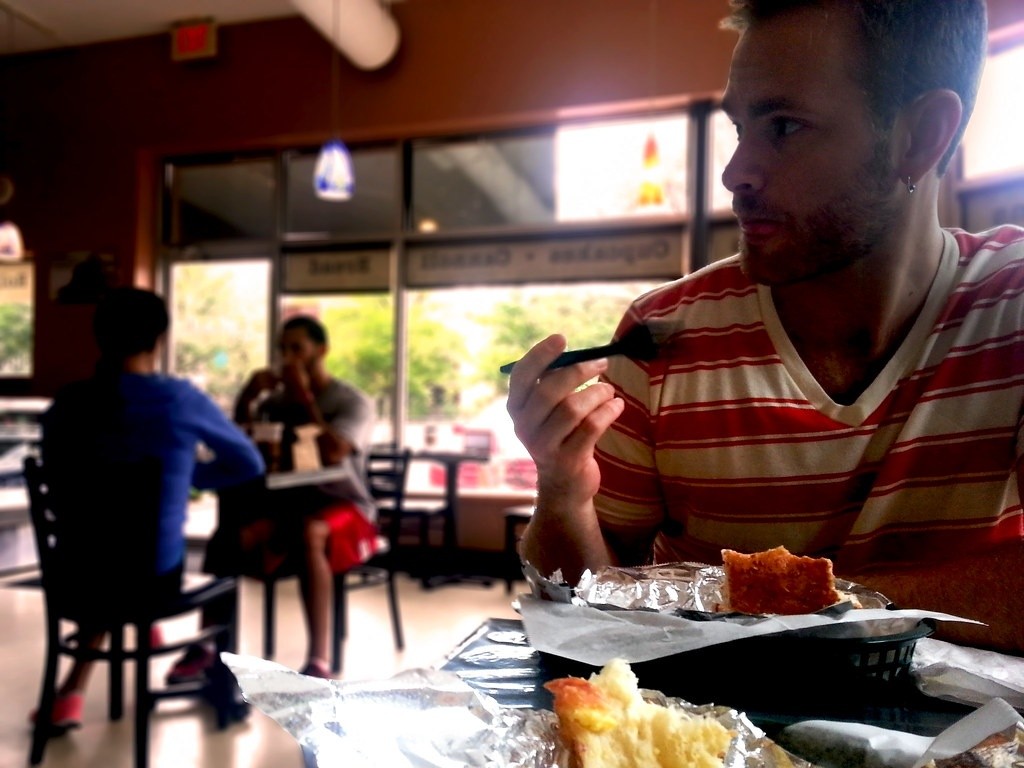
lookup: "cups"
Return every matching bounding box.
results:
[254,422,284,475]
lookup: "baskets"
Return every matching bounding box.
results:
[538,619,939,715]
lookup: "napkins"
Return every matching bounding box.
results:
[908,634,1024,710]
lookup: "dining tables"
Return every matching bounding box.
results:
[221,545,1024,768]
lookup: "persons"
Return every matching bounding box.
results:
[28,288,262,730]
[167,315,382,687]
[506,0,1024,664]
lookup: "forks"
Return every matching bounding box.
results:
[499,318,684,375]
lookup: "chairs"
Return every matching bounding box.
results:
[376,488,449,590]
[500,505,541,594]
[24,457,238,768]
[264,448,414,677]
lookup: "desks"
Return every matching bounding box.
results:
[415,447,486,590]
[190,466,349,721]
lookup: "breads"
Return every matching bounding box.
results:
[722,544,863,616]
[542,657,737,768]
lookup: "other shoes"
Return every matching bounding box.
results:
[167,641,216,683]
[300,662,333,681]
[31,690,82,729]
[147,625,162,647]
